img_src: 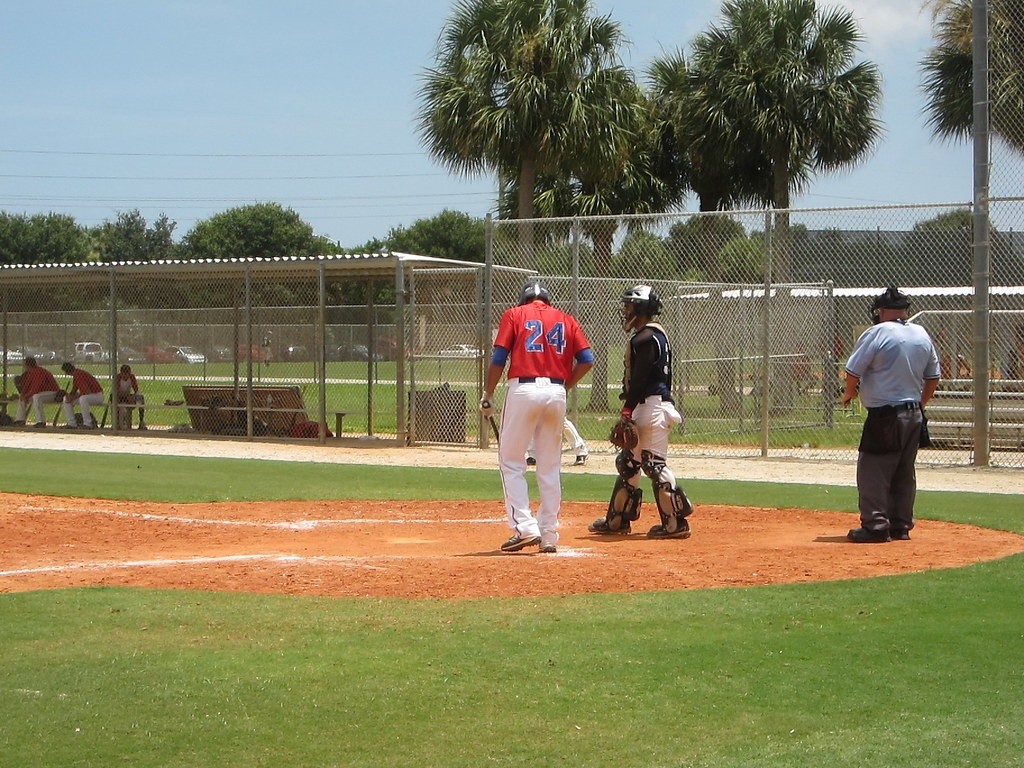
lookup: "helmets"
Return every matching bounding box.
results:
[619,285,662,332]
[520,282,551,305]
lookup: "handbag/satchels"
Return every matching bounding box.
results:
[858,406,900,454]
[919,402,930,449]
[289,421,334,438]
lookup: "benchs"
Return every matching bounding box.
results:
[0,400,355,438]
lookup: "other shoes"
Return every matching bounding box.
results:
[61,424,77,429]
[33,422,46,427]
[138,425,148,430]
[12,421,25,427]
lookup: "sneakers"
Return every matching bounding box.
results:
[890,527,910,540]
[501,535,542,552]
[526,457,536,465]
[574,455,589,466]
[538,541,556,552]
[588,521,632,534]
[847,528,890,542]
[648,520,691,538]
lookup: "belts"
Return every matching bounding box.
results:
[518,377,564,384]
[894,403,919,412]
[639,398,645,404]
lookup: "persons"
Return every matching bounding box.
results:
[773,341,811,413]
[110,365,146,429]
[1000,340,1024,390]
[589,285,692,539]
[61,363,103,429]
[943,343,971,391]
[841,289,941,542]
[12,357,60,428]
[526,418,590,466]
[479,282,594,553]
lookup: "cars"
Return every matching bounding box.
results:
[0,339,413,365]
[436,344,484,356]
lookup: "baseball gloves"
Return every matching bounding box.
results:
[610,419,638,449]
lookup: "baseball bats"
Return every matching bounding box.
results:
[291,385,309,427]
[482,400,499,442]
[100,397,110,429]
[26,403,32,417]
[52,380,70,427]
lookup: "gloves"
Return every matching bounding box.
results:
[480,391,495,417]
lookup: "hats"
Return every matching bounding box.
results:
[877,287,907,310]
